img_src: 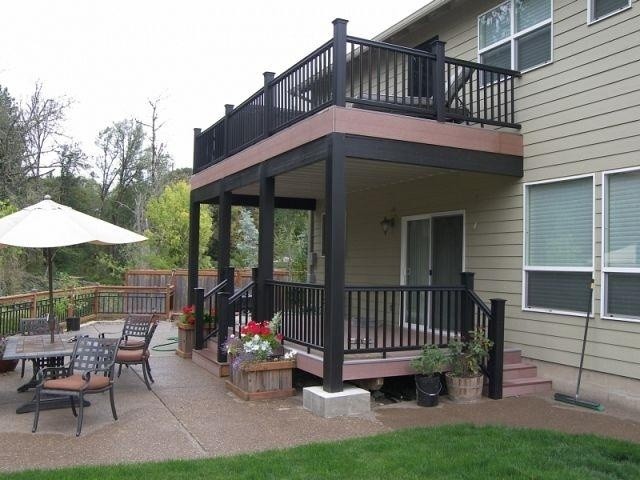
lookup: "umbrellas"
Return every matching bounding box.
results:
[1,198,150,345]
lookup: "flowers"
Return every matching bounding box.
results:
[219,311,299,373]
[177,301,217,325]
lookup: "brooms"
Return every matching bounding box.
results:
[554,279,605,412]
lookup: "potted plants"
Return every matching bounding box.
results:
[0,337,20,372]
[55,272,94,332]
[409,326,494,407]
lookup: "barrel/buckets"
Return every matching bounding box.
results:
[416,374,442,407]
[445,371,483,403]
[66,317,80,331]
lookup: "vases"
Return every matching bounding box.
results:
[225,356,297,401]
[174,318,218,359]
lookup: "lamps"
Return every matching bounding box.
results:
[379,216,395,238]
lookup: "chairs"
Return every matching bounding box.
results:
[351,62,479,126]
[2,315,160,437]
